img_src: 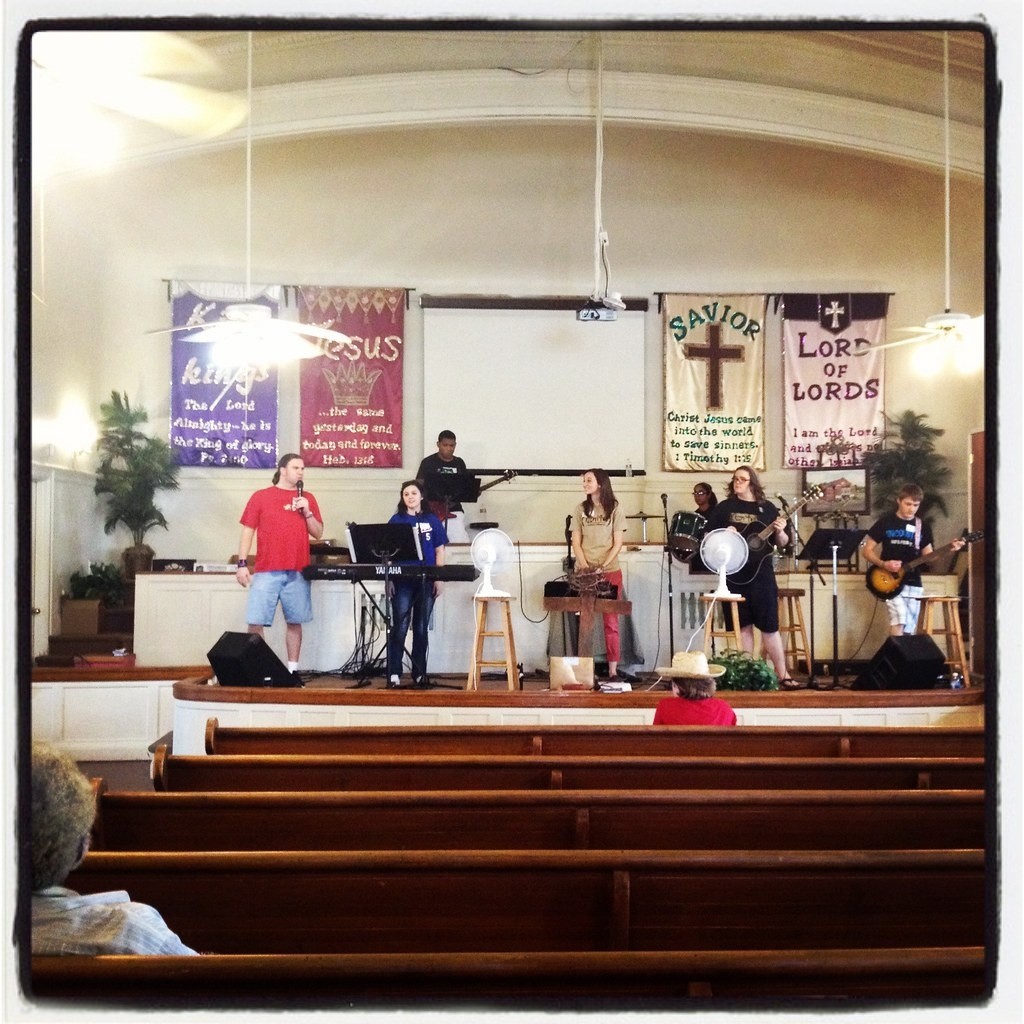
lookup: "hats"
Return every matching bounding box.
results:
[654,651,726,679]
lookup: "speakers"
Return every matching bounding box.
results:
[851,633,945,690]
[207,632,303,688]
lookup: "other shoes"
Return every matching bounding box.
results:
[386,674,400,688]
[416,676,430,687]
[292,670,305,688]
[610,675,624,683]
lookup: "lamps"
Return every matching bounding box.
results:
[148,37,357,355]
[851,31,984,355]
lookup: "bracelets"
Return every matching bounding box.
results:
[881,562,885,568]
[306,512,312,519]
[238,560,247,568]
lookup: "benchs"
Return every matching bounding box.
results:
[31,716,993,998]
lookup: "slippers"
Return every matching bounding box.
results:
[779,678,800,689]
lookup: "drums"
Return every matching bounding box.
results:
[668,509,708,550]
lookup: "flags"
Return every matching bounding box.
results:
[295,286,403,469]
[169,280,282,469]
[781,292,886,470]
[660,294,767,473]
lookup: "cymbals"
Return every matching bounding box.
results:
[624,510,665,519]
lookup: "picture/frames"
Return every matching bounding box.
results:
[802,464,868,517]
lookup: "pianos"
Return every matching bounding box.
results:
[299,563,481,690]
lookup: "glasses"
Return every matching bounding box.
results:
[692,491,707,496]
[731,477,750,483]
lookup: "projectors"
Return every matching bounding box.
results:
[575,308,618,322]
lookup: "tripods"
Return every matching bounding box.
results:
[397,571,462,690]
[775,499,868,691]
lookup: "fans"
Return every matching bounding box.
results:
[469,528,517,596]
[698,530,750,599]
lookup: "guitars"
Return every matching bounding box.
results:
[865,529,985,600]
[726,483,825,584]
[424,469,518,523]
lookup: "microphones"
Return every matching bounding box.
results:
[297,480,303,513]
[661,493,668,510]
[775,492,790,507]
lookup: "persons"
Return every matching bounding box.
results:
[415,430,470,543]
[652,651,737,727]
[385,479,450,685]
[688,482,718,575]
[236,453,324,688]
[703,465,800,689]
[862,483,966,636]
[571,468,628,683]
[30,739,197,957]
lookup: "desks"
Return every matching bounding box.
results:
[763,572,958,667]
[132,573,554,680]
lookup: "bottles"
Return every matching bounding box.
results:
[949,664,964,689]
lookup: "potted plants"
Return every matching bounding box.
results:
[93,389,180,571]
[61,565,127,633]
[862,409,949,572]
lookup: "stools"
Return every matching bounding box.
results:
[698,596,748,664]
[463,597,519,690]
[911,596,971,687]
[773,589,812,677]
